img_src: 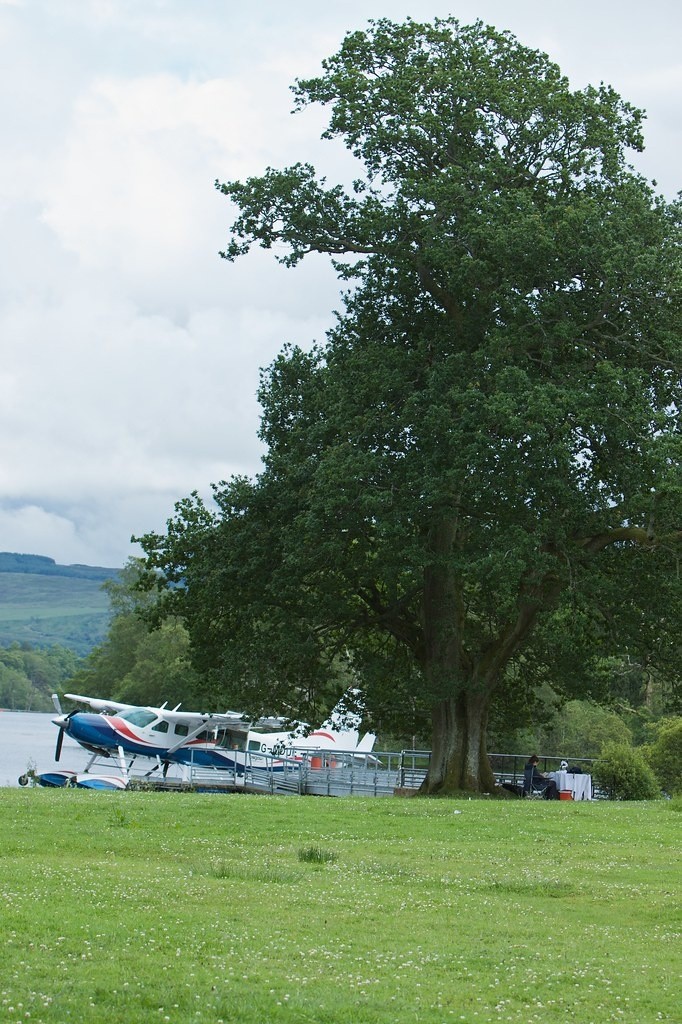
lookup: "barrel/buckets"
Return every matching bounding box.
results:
[311,755,335,770]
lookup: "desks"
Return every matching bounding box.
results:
[550,772,592,800]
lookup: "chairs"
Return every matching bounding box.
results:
[524,763,552,801]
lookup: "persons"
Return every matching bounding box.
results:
[525,755,560,801]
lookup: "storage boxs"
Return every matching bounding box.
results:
[557,790,572,800]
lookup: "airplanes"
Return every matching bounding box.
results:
[51,688,383,777]
